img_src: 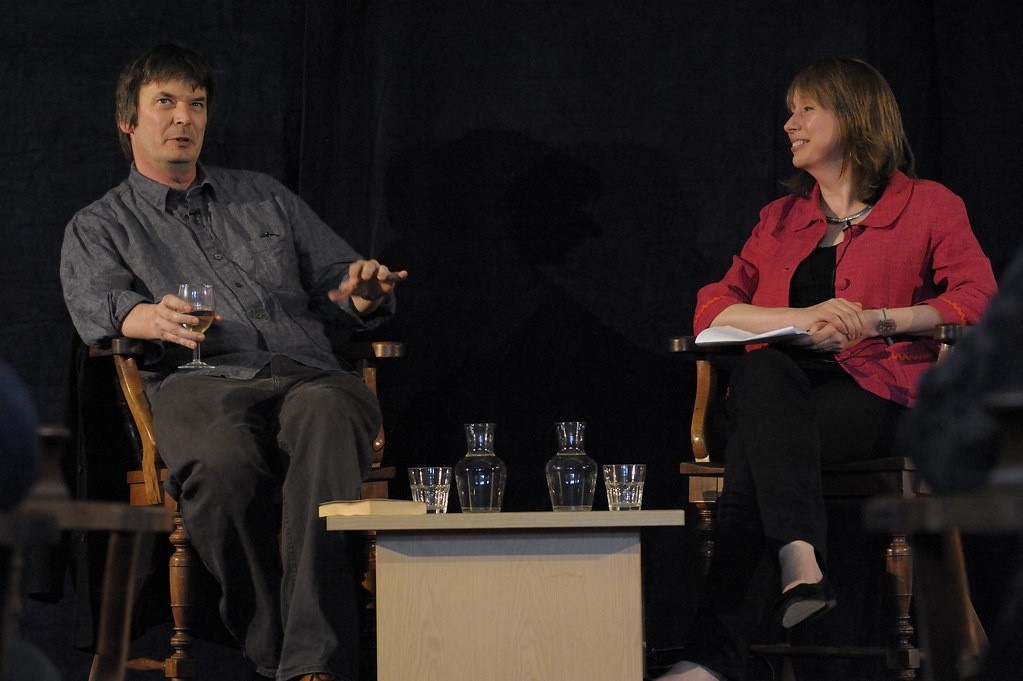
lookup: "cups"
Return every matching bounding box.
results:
[407,467,452,514]
[603,464,646,511]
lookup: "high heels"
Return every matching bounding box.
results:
[781,557,838,629]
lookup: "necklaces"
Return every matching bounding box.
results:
[825,203,873,224]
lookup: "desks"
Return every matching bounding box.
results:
[322,500,691,681]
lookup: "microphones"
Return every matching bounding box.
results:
[186,208,202,217]
[847,220,853,229]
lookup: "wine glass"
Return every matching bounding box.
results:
[177,283,217,369]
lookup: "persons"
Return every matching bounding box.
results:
[649,54,998,681]
[60,43,407,681]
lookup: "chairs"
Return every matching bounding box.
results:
[660,325,975,681]
[90,334,405,681]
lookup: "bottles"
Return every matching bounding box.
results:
[545,422,597,512]
[454,422,507,513]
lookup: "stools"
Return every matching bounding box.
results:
[0,497,175,681]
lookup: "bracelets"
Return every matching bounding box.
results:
[875,306,897,338]
